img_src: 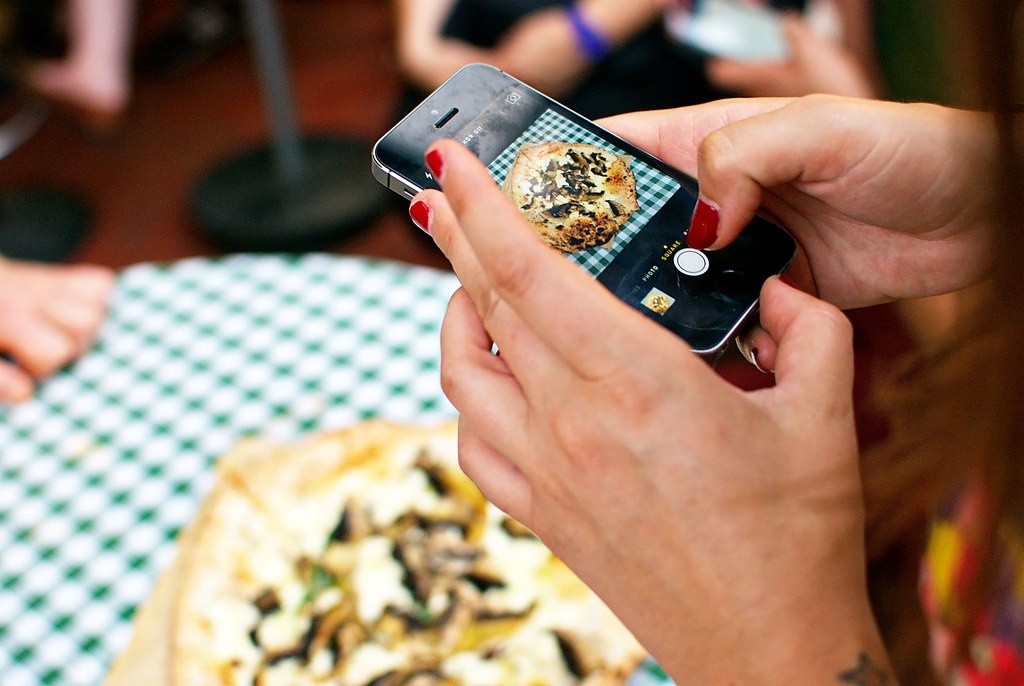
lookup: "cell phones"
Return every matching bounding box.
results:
[370,61,797,360]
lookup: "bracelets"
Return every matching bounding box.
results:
[560,1,613,62]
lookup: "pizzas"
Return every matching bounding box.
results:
[502,141,638,253]
[165,421,653,685]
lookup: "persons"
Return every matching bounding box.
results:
[0,0,1024,683]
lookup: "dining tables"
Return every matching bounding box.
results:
[0,253,676,686]
